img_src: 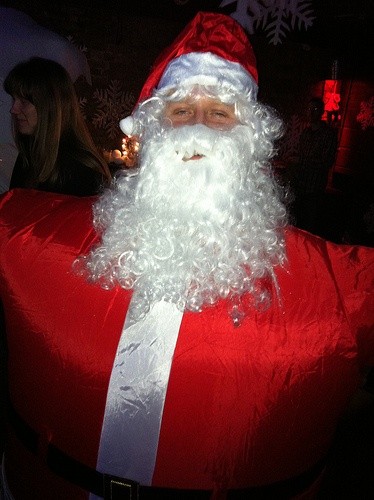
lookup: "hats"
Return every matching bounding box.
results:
[118,12,261,135]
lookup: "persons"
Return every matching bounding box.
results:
[283,91,374,248]
[3,57,114,199]
[0,12,374,498]
